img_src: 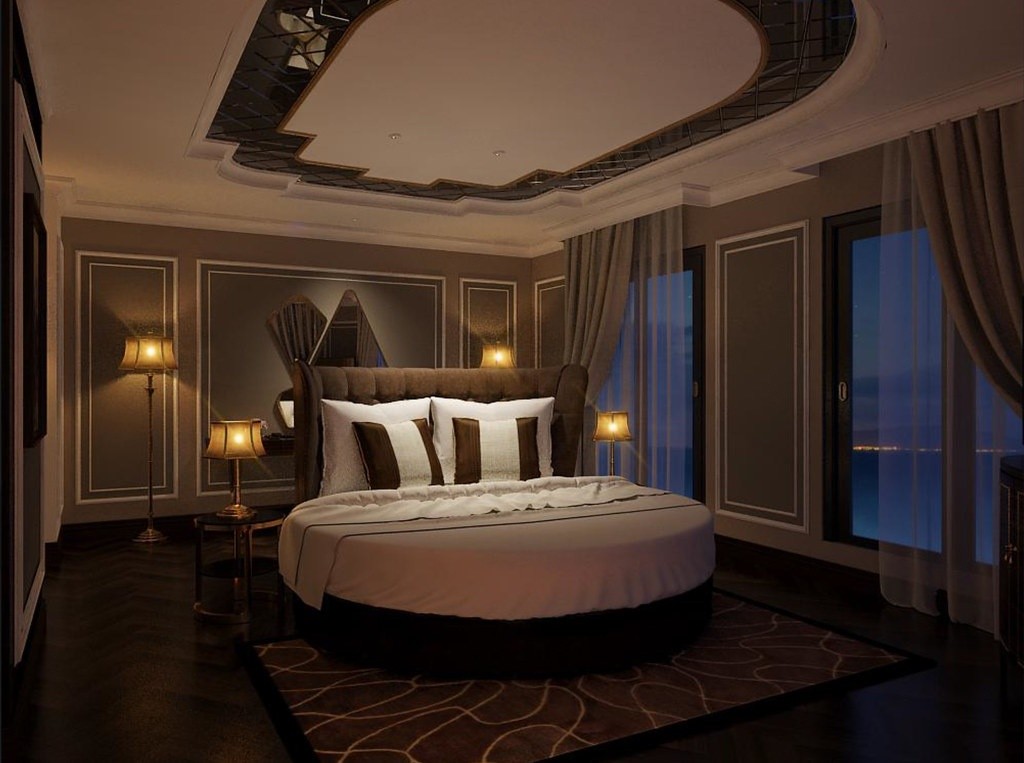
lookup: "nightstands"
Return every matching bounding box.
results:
[193,504,288,622]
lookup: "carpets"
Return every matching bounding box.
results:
[234,586,943,763]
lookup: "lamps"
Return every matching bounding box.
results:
[117,335,179,544]
[479,344,518,369]
[202,418,268,522]
[592,409,635,477]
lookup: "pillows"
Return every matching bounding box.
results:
[350,419,445,491]
[317,396,436,498]
[429,395,555,486]
[451,417,542,485]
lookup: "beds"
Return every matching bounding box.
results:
[278,360,717,678]
